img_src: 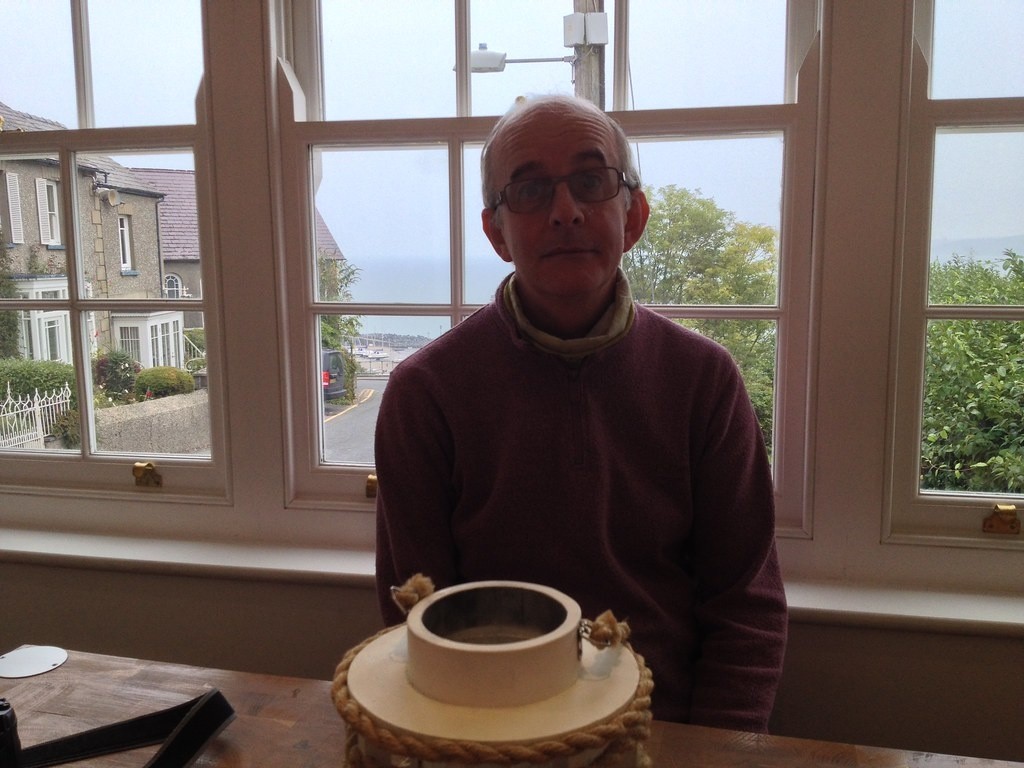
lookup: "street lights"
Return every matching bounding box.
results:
[453,0,606,110]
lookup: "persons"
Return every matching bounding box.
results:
[374,91,793,735]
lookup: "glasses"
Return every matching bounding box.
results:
[491,166,633,215]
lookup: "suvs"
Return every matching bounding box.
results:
[322,347,347,400]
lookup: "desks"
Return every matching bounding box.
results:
[0,637,1024,768]
